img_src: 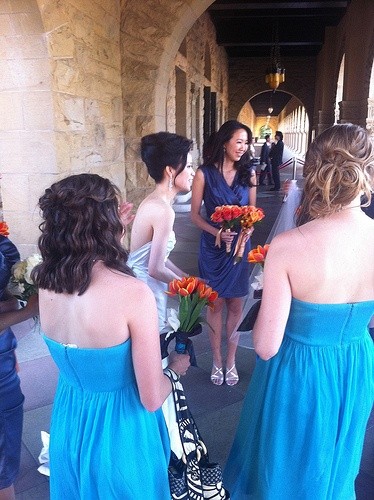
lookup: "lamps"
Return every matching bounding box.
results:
[264,9,286,96]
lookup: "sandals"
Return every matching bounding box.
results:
[210,362,224,385]
[225,363,239,386]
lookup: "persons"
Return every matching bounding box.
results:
[191,120,260,386]
[258,131,285,191]
[31,173,192,500]
[125,132,210,369]
[222,123,374,500]
[0,221,40,500]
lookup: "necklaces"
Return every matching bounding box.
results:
[338,204,362,211]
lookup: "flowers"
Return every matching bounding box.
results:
[164,275,218,358]
[7,253,43,335]
[0,221,9,238]
[209,203,271,269]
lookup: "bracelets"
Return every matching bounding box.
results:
[187,272,191,278]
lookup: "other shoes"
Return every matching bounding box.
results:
[269,187,280,191]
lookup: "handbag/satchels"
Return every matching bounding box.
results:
[163,367,230,500]
